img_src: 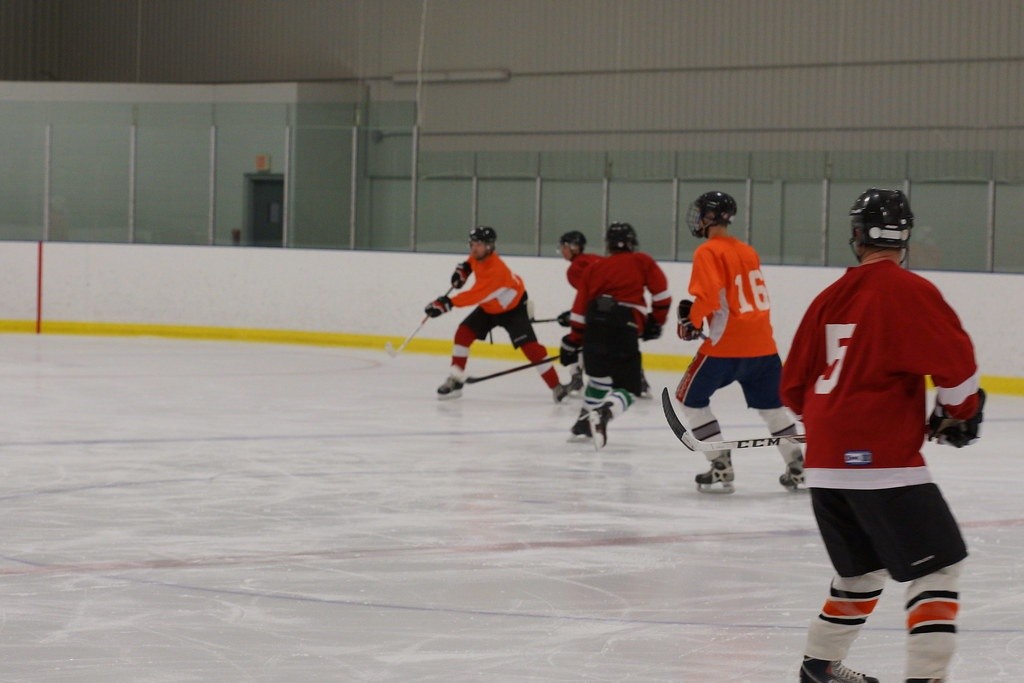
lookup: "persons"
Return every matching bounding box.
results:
[675,191,807,490]
[779,187,987,683]
[426,226,565,405]
[567,222,672,449]
[557,230,648,391]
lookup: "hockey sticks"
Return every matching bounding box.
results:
[659,386,970,455]
[465,333,645,384]
[699,332,706,340]
[527,300,564,323]
[384,285,455,359]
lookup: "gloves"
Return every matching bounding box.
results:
[678,300,702,340]
[560,335,579,366]
[643,314,660,340]
[451,263,472,288]
[558,311,570,325]
[929,390,986,448]
[425,296,453,316]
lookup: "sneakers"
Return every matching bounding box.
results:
[554,386,570,404]
[437,378,463,398]
[588,407,611,449]
[800,656,878,683]
[779,456,806,491]
[640,371,653,399]
[567,374,583,397]
[695,451,735,493]
[569,418,591,443]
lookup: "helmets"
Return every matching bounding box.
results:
[606,222,638,245]
[849,187,914,248]
[687,191,737,238]
[560,230,587,244]
[468,227,496,241]
